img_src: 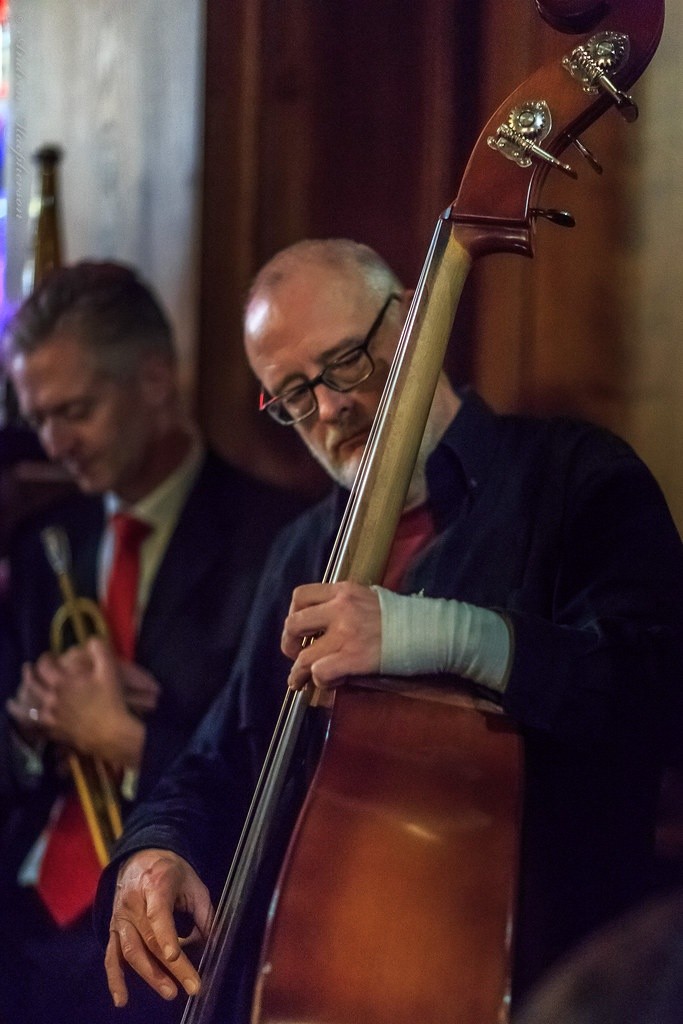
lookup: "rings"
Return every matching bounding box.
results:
[29,707,39,722]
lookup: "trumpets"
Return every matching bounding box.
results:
[44,530,138,870]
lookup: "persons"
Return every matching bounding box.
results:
[0,256,307,1024]
[94,238,683,1024]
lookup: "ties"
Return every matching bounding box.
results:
[36,515,155,929]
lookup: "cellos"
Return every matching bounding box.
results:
[177,2,666,1024]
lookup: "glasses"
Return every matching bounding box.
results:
[259,293,402,425]
